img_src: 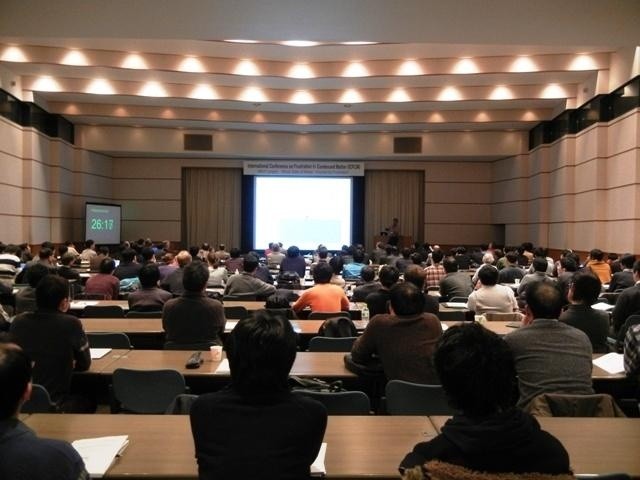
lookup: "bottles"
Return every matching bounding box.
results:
[362,303,370,326]
[235,269,240,275]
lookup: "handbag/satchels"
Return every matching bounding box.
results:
[286,376,346,391]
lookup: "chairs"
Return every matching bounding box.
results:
[1,261,640,480]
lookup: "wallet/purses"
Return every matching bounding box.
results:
[185,352,202,369]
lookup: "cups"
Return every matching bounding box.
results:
[515,278,520,286]
[474,315,485,326]
[210,345,223,362]
[300,278,305,285]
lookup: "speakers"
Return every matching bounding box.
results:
[393,137,422,154]
[184,134,212,151]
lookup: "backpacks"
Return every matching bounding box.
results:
[318,317,357,337]
